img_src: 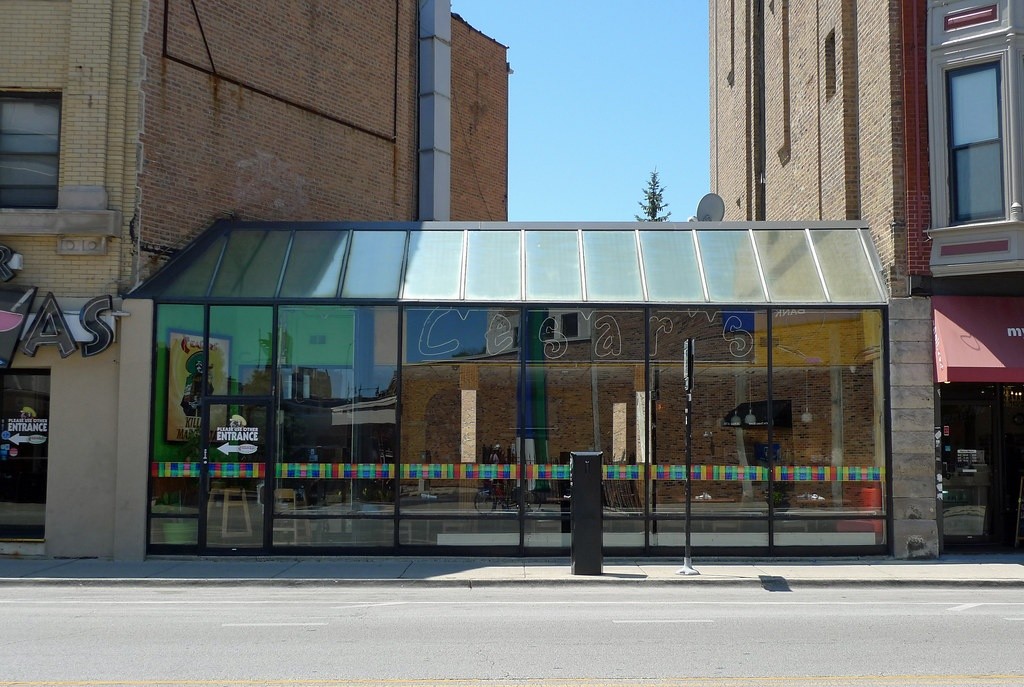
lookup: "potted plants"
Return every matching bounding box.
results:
[772,490,790,512]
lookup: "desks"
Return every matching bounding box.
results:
[207,488,253,539]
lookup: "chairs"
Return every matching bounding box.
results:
[273,487,298,543]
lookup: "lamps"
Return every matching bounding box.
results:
[705,376,726,428]
[801,368,814,423]
[730,373,741,426]
[745,369,757,425]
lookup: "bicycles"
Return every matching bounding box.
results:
[474,478,541,513]
